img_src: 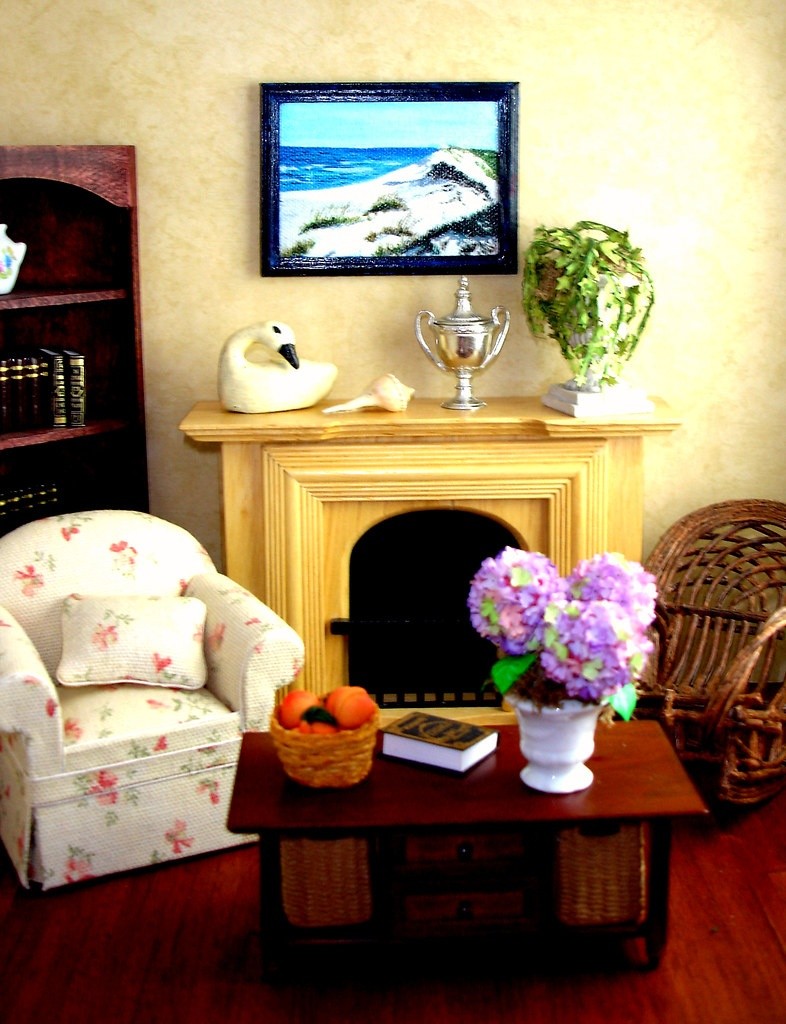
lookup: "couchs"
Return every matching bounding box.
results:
[0,510,305,890]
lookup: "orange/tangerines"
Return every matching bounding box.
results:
[278,684,377,736]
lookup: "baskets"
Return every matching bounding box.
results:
[553,823,643,925]
[280,833,372,928]
[271,694,380,787]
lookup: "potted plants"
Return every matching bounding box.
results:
[521,220,655,392]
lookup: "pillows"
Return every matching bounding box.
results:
[56,592,208,690]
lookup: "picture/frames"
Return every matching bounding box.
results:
[259,82,519,278]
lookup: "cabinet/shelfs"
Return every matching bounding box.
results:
[0,145,150,537]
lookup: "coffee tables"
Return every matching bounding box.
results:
[227,720,709,990]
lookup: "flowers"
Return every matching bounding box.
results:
[468,546,659,728]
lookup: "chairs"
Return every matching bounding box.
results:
[632,498,786,805]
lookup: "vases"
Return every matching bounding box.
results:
[503,692,605,793]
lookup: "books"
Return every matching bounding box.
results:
[0,346,85,539]
[378,712,499,777]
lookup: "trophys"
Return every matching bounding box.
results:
[415,275,510,411]
